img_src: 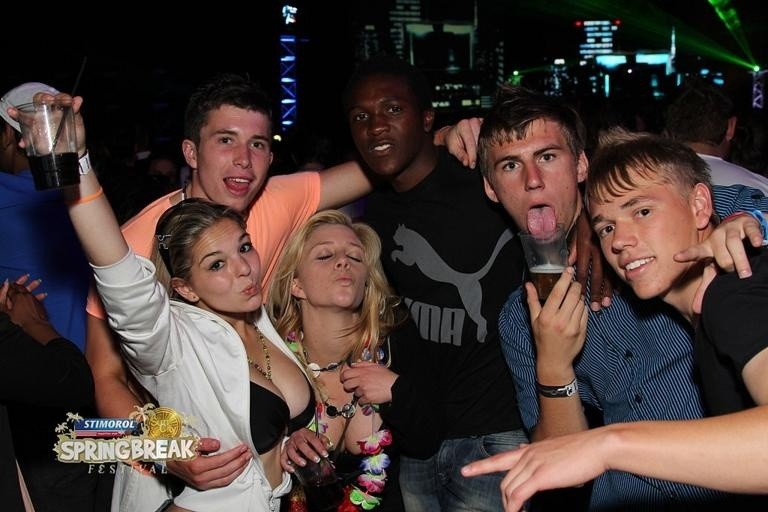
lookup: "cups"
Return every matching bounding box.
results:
[519,226,573,302]
[288,447,345,512]
[14,100,82,194]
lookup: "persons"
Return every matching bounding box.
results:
[0,56,768,512]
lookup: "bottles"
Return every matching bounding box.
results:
[428,83,484,109]
